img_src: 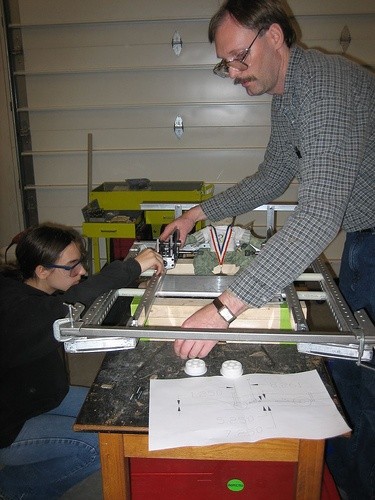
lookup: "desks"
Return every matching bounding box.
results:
[73,316,352,500]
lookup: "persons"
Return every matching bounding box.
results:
[1,225,164,500]
[157,1,374,500]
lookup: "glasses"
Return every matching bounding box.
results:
[213,28,263,78]
[42,258,85,277]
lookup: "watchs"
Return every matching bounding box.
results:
[212,297,237,323]
